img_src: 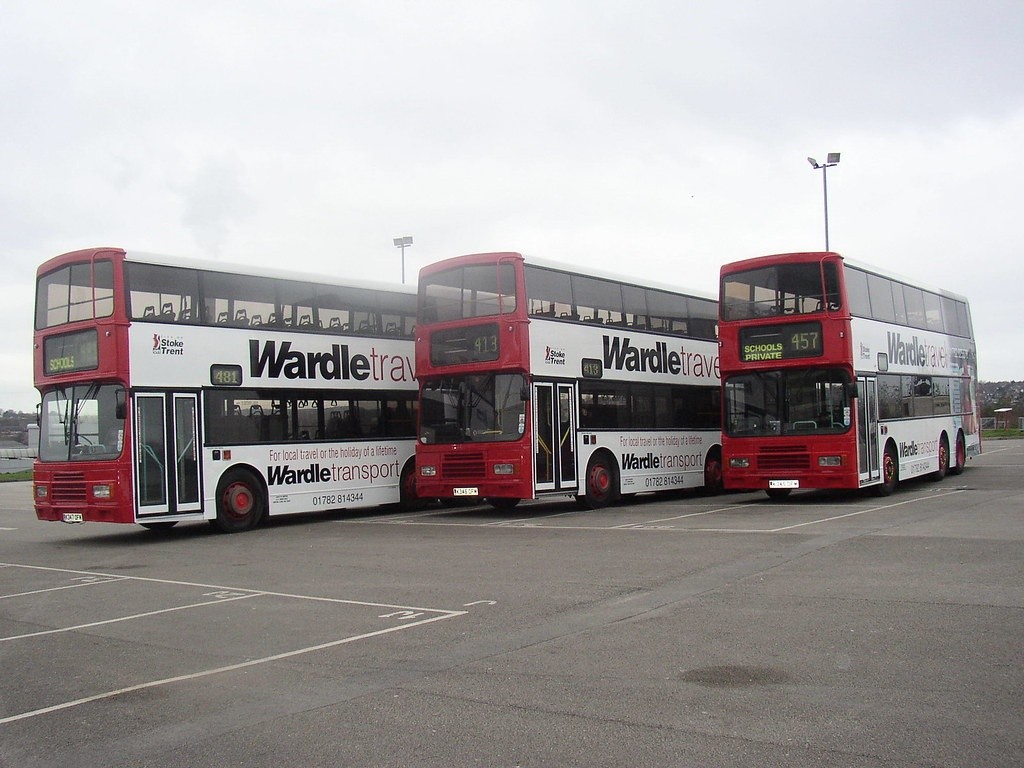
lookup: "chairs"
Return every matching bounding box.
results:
[535,309,688,335]
[784,308,797,314]
[770,306,783,315]
[326,410,352,439]
[224,405,288,442]
[143,303,415,339]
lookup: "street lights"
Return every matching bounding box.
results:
[393,235,413,282]
[807,152,842,253]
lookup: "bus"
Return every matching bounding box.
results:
[27,245,549,536]
[406,250,824,516]
[705,252,982,503]
